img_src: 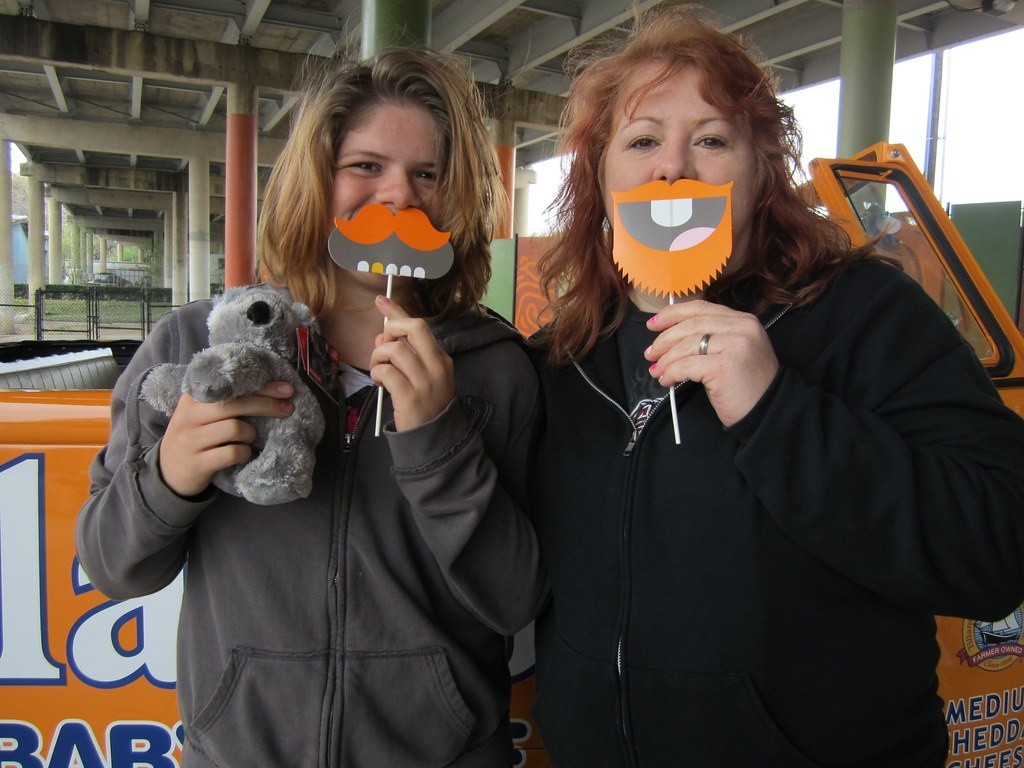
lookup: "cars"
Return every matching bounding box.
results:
[86,271,133,289]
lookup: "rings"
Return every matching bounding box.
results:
[698,333,712,355]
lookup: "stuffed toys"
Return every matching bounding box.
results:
[138,280,324,506]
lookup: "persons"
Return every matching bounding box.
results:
[525,7,1024,768]
[73,48,550,768]
